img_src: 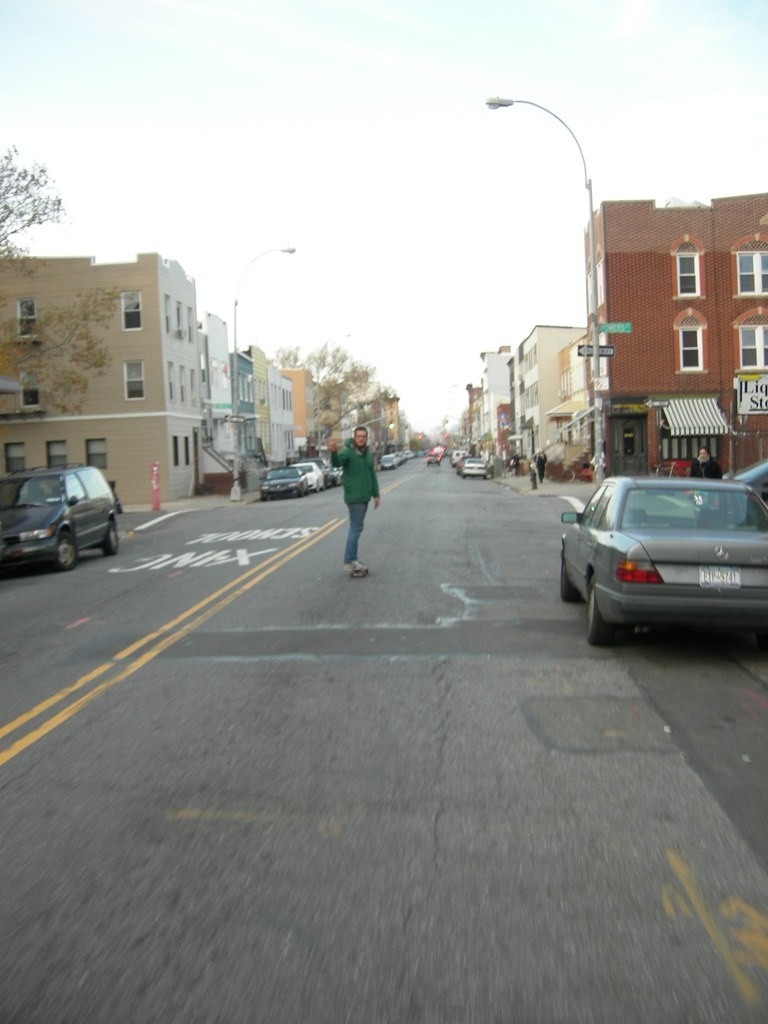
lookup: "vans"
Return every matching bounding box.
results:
[451,451,470,467]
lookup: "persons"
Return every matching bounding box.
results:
[690,447,722,480]
[327,426,381,577]
[534,448,547,483]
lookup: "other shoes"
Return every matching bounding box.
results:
[344,560,369,570]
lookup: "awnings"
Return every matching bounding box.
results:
[663,398,728,436]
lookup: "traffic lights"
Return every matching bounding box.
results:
[389,420,394,429]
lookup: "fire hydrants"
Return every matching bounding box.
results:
[530,470,536,489]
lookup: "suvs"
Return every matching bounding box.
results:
[0,462,119,573]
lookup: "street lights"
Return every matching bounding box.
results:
[488,98,603,485]
[231,248,296,501]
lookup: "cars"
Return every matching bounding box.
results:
[461,459,486,480]
[287,462,325,491]
[458,455,480,474]
[260,468,308,501]
[426,453,441,465]
[560,475,768,647]
[694,457,768,526]
[297,459,344,488]
[380,450,415,470]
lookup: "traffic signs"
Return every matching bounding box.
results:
[579,345,614,357]
